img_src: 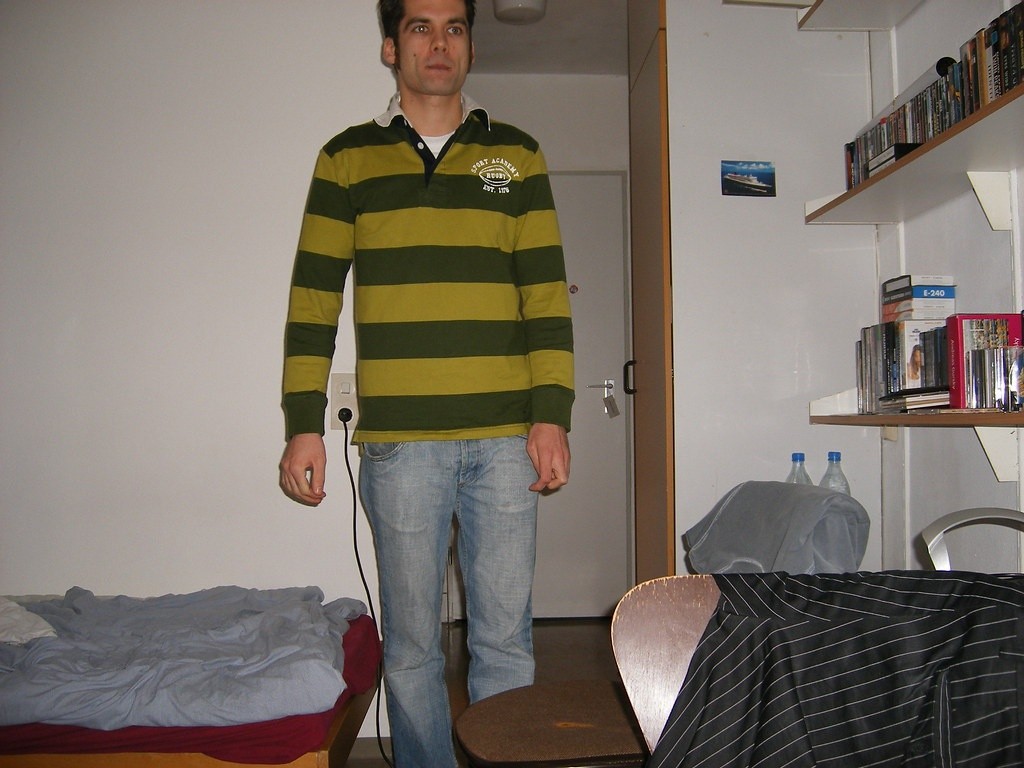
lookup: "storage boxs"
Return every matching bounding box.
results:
[948,314,1023,409]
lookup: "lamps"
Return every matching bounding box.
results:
[493,0,546,25]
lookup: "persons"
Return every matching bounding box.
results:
[907,346,921,379]
[278,0,575,768]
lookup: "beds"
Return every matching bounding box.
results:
[0,586,385,768]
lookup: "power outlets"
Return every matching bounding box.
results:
[331,373,360,431]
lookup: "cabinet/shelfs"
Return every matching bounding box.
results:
[798,2,1024,425]
[623,0,886,584]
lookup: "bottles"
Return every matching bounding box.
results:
[817,452,850,496]
[784,453,814,485]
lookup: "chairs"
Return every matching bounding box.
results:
[455,479,872,768]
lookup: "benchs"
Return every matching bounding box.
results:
[610,570,1024,768]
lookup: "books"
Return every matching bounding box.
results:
[844,0,1024,418]
